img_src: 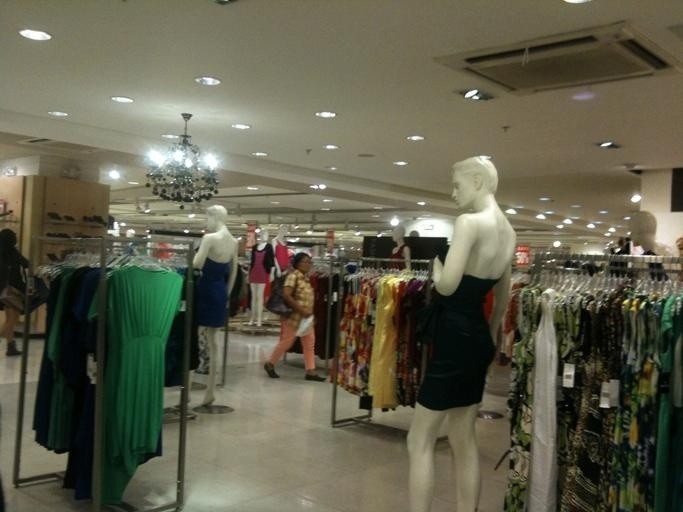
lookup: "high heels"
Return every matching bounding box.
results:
[111,216,114,229]
[108,216,111,230]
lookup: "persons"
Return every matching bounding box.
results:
[0,228,29,357]
[264,224,290,325]
[263,252,328,382]
[406,154,516,512]
[629,211,678,282]
[173,204,237,407]
[247,228,275,328]
[391,226,411,270]
[124,229,140,257]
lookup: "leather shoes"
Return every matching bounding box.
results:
[56,232,64,237]
[52,253,58,261]
[64,215,69,220]
[305,374,326,382]
[65,233,72,238]
[47,253,55,261]
[47,233,53,236]
[263,362,280,378]
[89,216,93,221]
[82,234,87,238]
[53,212,61,220]
[83,216,88,221]
[68,215,74,221]
[48,212,55,219]
[53,232,57,235]
[94,216,99,220]
[95,234,102,238]
[88,235,92,238]
[75,234,82,238]
[98,215,103,223]
[5,343,25,359]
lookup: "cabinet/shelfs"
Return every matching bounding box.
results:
[0,176,111,337]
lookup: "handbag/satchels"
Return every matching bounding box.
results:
[22,264,50,313]
[416,257,439,344]
[266,273,298,317]
[2,252,27,315]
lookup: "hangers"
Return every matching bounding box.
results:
[309,262,340,283]
[506,250,683,324]
[29,248,189,292]
[342,266,429,295]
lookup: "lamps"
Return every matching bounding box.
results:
[142,112,219,209]
